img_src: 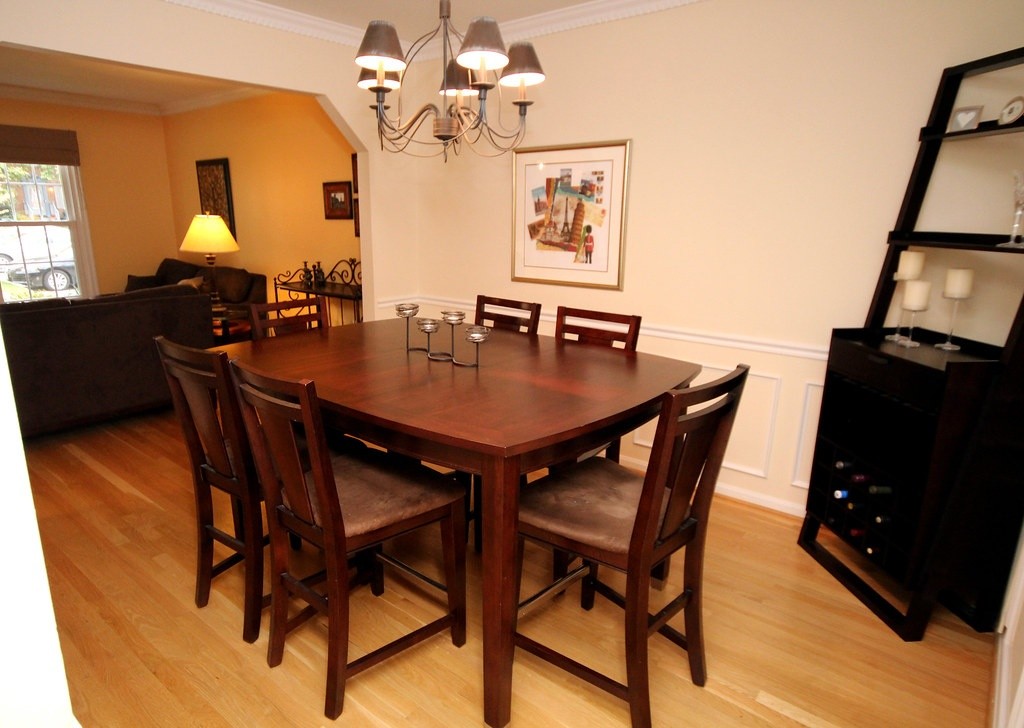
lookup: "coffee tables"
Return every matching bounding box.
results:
[212,319,252,337]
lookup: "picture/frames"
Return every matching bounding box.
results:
[352,153,358,193]
[353,198,360,237]
[323,181,353,220]
[195,157,237,243]
[510,137,632,292]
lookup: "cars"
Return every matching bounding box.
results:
[0,217,79,292]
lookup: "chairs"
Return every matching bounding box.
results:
[554,305,643,595]
[247,296,328,340]
[512,364,752,728]
[229,357,467,721]
[153,334,370,646]
[440,294,544,556]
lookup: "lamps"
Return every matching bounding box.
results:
[179,210,240,328]
[356,0,545,166]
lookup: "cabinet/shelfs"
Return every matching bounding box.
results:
[797,47,1024,642]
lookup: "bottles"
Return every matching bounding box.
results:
[305,270,313,289]
[828,455,902,560]
[316,268,325,287]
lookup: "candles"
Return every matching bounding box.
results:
[944,267,975,299]
[898,250,926,280]
[903,279,931,310]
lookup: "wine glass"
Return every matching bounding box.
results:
[997,173,1024,250]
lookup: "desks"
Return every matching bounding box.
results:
[275,257,362,328]
[204,315,701,728]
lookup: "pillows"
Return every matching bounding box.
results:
[213,267,254,303]
[175,276,204,290]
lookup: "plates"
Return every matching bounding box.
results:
[997,96,1024,125]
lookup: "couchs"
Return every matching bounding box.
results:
[96,256,267,346]
[0,283,214,442]
